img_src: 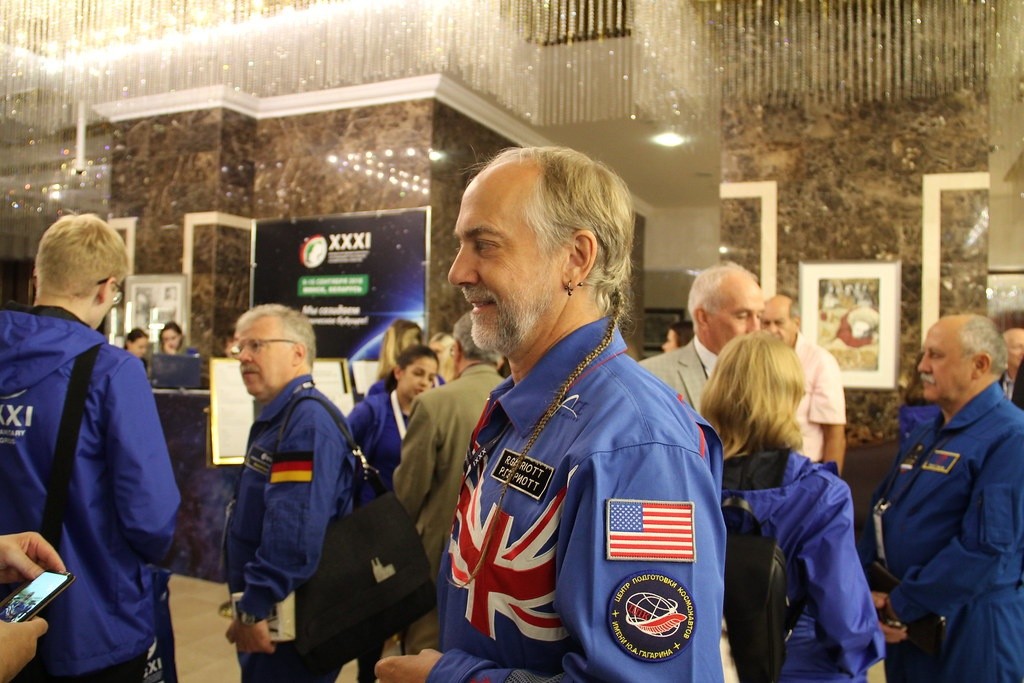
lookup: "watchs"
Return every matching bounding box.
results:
[237,610,264,624]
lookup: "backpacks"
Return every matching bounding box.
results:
[721,498,813,683]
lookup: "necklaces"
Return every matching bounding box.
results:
[454,419,510,491]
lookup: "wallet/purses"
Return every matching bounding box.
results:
[869,560,944,657]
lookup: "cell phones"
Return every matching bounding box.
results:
[0,568,75,622]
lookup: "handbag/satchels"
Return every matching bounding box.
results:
[278,397,442,676]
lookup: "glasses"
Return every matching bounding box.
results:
[229,335,298,359]
[98,275,124,305]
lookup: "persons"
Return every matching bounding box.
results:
[0,532,66,683]
[121,328,149,372]
[637,258,891,683]
[225,303,365,683]
[345,144,728,683]
[0,591,35,621]
[0,212,183,683]
[854,313,1024,683]
[158,320,200,358]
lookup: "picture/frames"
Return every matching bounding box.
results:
[796,257,902,391]
